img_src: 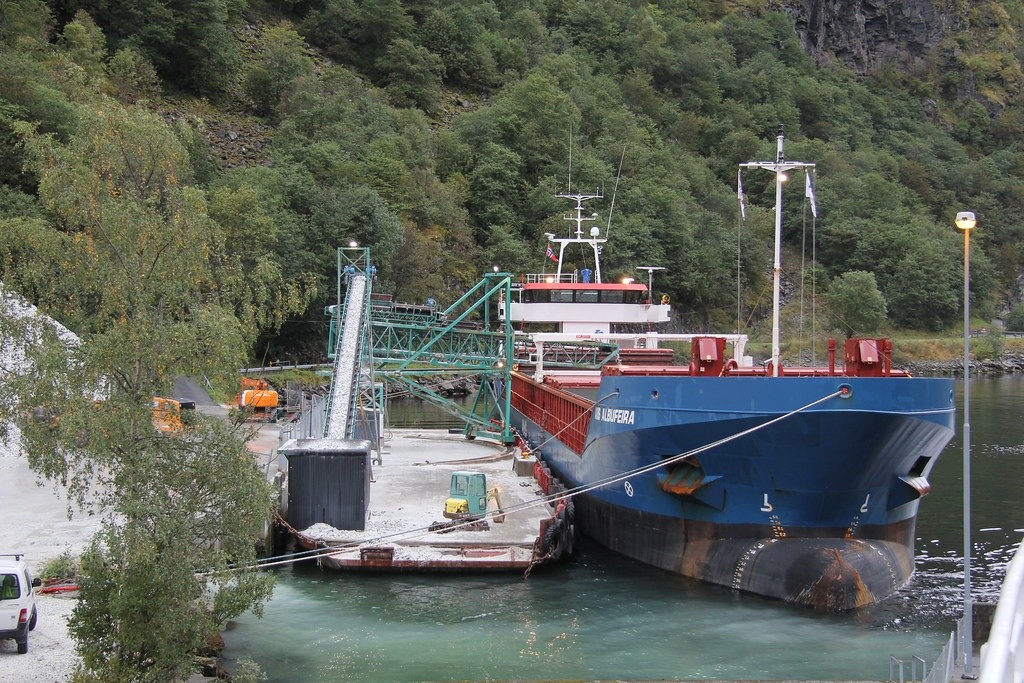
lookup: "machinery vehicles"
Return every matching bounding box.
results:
[236,375,280,421]
[47,396,183,449]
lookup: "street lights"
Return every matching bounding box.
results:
[953,209,979,682]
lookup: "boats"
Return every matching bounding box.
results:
[491,123,957,609]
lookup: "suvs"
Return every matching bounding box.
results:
[0,554,43,654]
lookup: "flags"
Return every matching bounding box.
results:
[546,244,558,263]
